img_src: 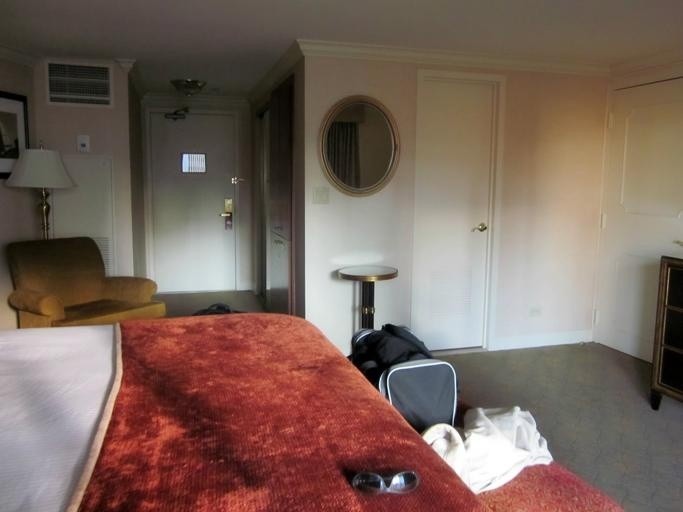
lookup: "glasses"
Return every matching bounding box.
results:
[351,469,421,497]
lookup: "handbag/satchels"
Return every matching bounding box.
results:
[346,323,459,432]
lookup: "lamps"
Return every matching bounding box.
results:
[4,142,78,242]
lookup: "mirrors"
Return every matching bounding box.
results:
[315,94,402,198]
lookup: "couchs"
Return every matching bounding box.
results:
[0,235,169,328]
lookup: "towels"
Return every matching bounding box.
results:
[417,395,551,496]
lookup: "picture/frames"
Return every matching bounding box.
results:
[0,90,34,180]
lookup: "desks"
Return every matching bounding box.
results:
[337,260,400,362]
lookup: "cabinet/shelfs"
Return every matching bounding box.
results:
[650,255,683,413]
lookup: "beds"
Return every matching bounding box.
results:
[0,310,495,512]
